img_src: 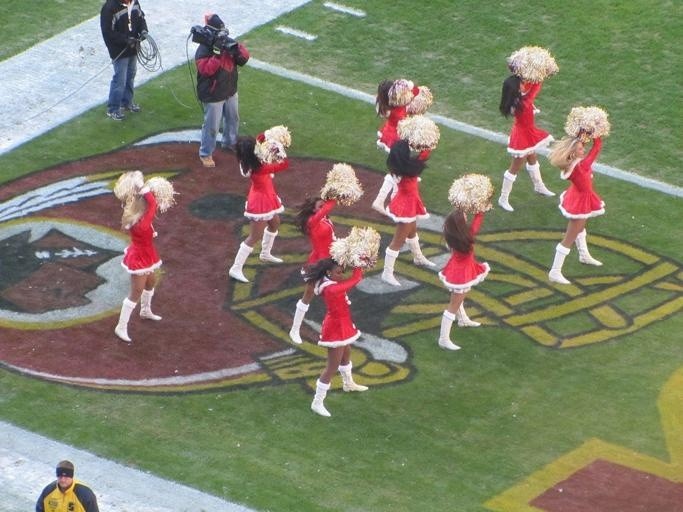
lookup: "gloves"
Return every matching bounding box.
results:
[212,37,235,56]
[127,31,147,50]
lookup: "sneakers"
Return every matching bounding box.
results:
[200,155,215,168]
[120,104,139,112]
[105,110,126,120]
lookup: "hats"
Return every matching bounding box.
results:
[56,460,74,478]
[208,15,225,32]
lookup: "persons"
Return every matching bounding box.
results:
[288,197,336,344]
[100,0,149,120]
[195,13,249,168]
[381,139,436,287]
[371,80,419,216]
[304,258,369,417]
[438,208,491,352]
[35,460,99,512]
[114,186,163,342]
[547,128,605,285]
[498,75,556,212]
[228,132,290,284]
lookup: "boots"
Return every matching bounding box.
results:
[338,361,368,393]
[259,227,285,262]
[372,174,394,215]
[114,298,138,342]
[406,235,435,266]
[526,161,554,195]
[310,378,332,417]
[382,245,403,287]
[289,300,310,343]
[499,171,517,211]
[229,242,254,282]
[139,287,161,321]
[438,302,480,350]
[549,229,602,285]
